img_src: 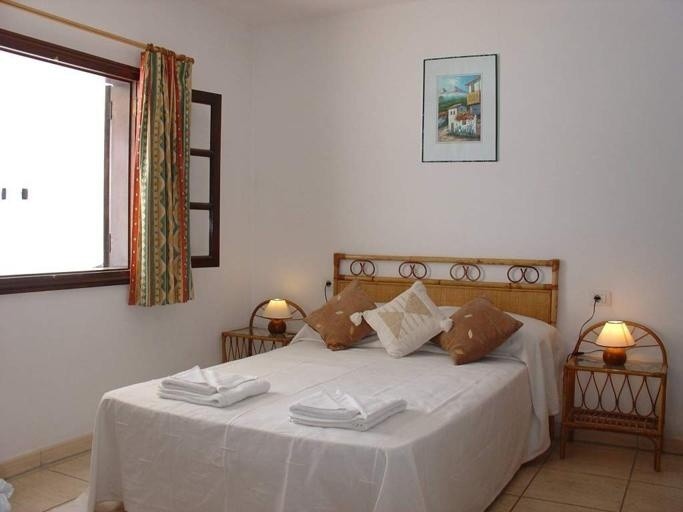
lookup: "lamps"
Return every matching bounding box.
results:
[595,321,636,366]
[262,299,292,334]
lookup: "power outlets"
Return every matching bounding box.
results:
[592,290,611,306]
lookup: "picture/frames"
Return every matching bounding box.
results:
[422,54,498,162]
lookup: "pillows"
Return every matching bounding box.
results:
[295,278,557,366]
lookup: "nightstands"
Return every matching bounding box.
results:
[559,320,668,472]
[221,298,308,363]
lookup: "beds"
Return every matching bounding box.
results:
[103,252,559,512]
